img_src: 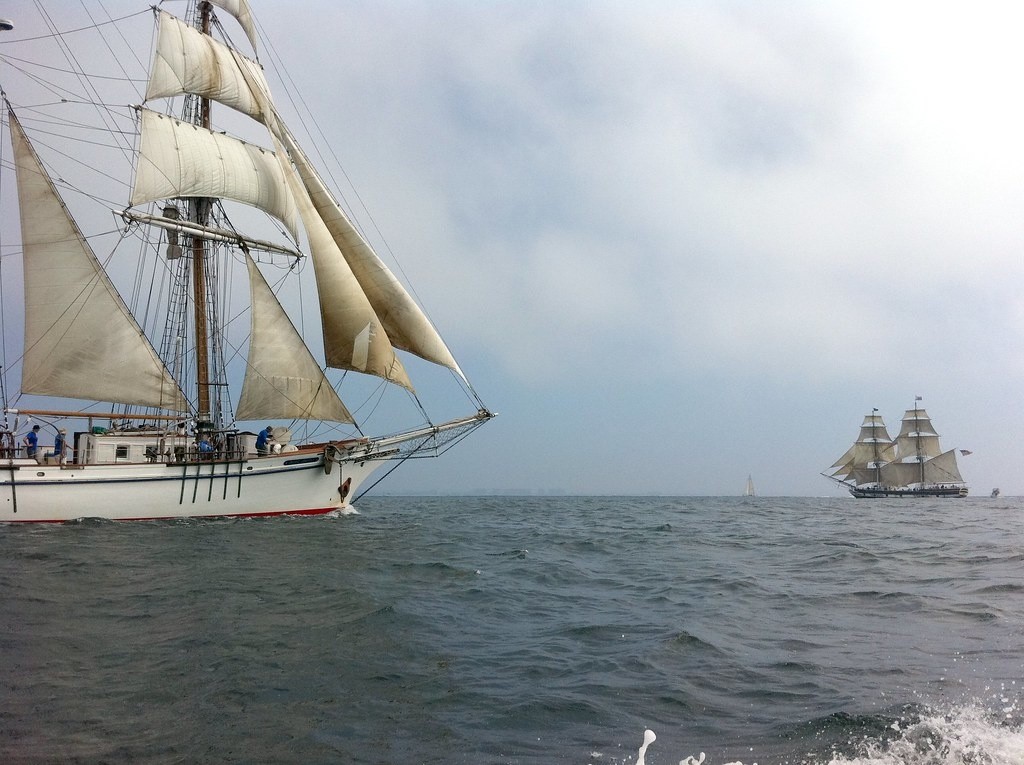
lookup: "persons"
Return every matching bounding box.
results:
[23,425,40,459]
[190,440,198,461]
[255,426,276,458]
[54,428,73,465]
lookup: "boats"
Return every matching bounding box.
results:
[990,487,1002,498]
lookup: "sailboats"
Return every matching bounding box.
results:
[817,394,973,498]
[0,3,499,524]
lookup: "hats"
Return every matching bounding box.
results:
[266,426,273,431]
[58,428,67,435]
[32,425,41,429]
